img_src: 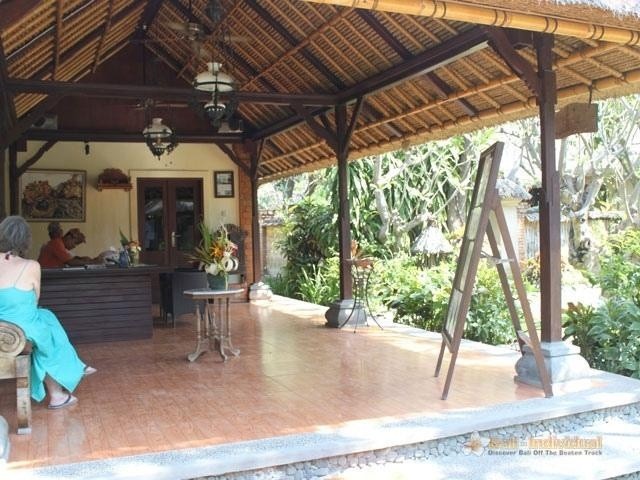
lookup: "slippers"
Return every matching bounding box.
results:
[47,395,77,409]
[84,365,98,376]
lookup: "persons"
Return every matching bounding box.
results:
[37,227,104,267]
[0,214,100,411]
[40,222,76,267]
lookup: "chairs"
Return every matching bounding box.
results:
[0,319,35,436]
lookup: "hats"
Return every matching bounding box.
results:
[69,227,87,244]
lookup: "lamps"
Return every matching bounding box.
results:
[132,2,241,162]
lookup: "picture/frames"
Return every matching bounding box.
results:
[15,166,88,224]
[214,170,235,199]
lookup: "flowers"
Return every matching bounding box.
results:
[187,214,238,291]
[118,227,142,256]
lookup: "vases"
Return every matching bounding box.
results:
[207,273,230,291]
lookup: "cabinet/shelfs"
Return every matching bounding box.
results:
[159,270,207,329]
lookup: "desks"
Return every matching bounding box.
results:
[183,286,246,364]
